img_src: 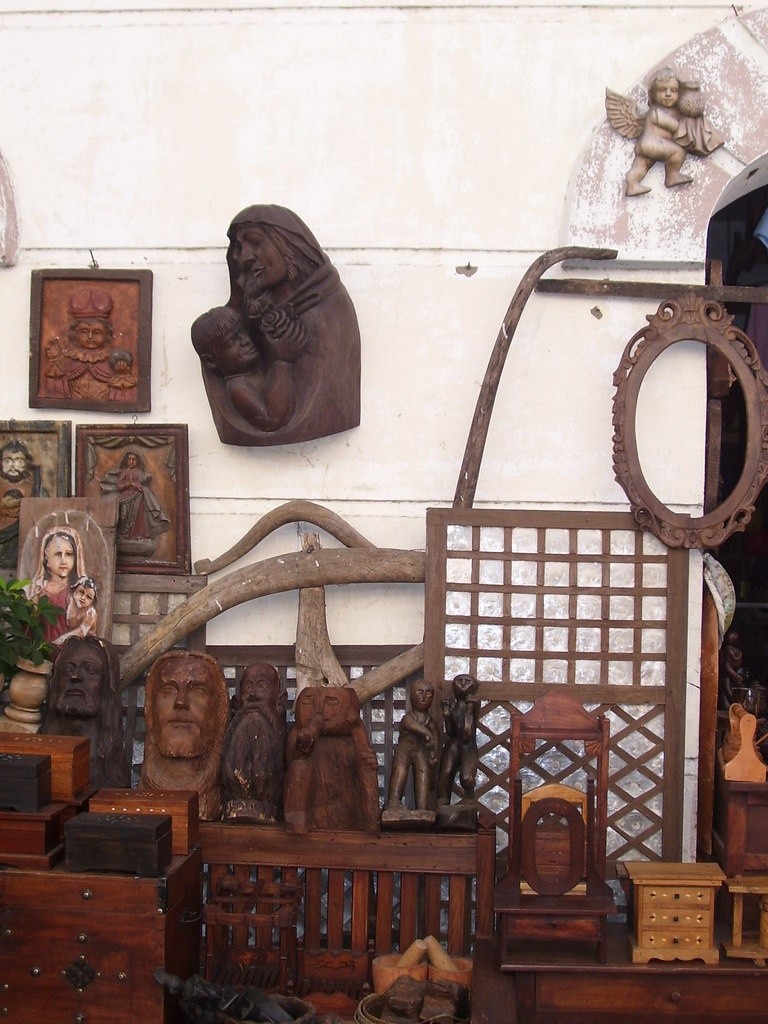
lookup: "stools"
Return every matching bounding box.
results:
[198,875,301,997]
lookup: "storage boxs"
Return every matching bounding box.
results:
[713,762,768,877]
[0,732,200,1024]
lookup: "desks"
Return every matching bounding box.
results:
[497,923,768,1024]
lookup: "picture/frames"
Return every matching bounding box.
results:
[73,422,191,575]
[0,418,73,573]
[27,268,154,413]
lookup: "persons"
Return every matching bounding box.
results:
[189,205,362,447]
[40,288,138,400]
[283,688,381,836]
[40,635,130,788]
[222,661,287,825]
[626,69,692,196]
[22,530,97,645]
[99,448,170,542]
[386,679,439,812]
[438,673,481,799]
[139,650,228,821]
[0,443,53,568]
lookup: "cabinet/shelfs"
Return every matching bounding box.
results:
[626,862,725,966]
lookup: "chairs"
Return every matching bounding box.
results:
[489,690,619,967]
